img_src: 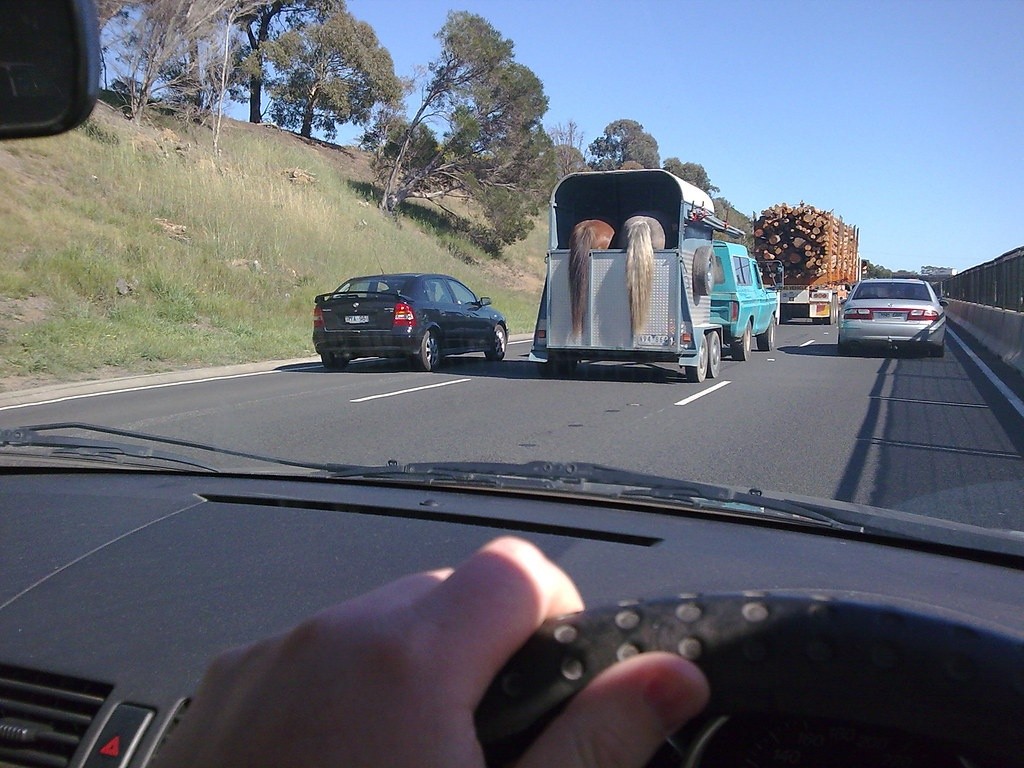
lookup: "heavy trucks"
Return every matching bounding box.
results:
[753,210,867,325]
[527,168,785,384]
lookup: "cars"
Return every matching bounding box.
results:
[312,272,508,371]
[837,277,949,356]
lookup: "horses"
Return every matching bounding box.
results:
[567,215,665,337]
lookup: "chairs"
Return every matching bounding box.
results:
[378,290,398,298]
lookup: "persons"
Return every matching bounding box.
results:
[160,538,713,768]
